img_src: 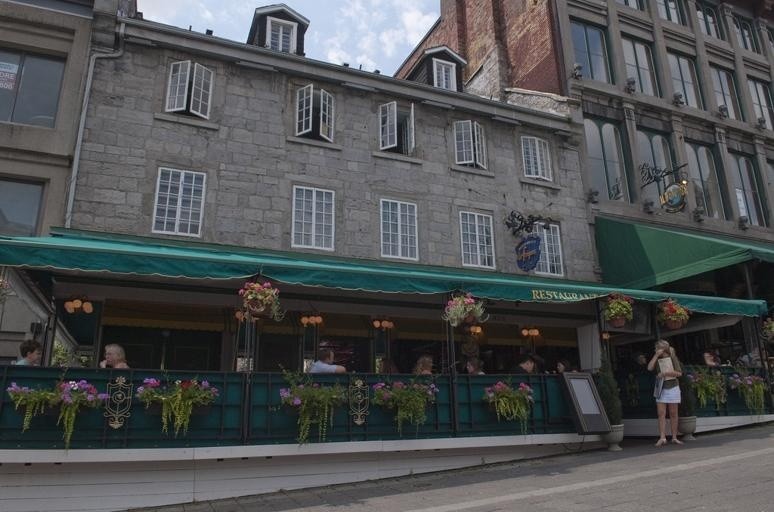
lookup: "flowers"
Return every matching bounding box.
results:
[8,381,58,434]
[279,375,347,446]
[55,380,107,450]
[655,297,691,328]
[371,382,440,439]
[483,381,535,441]
[441,291,490,327]
[685,370,767,416]
[237,281,289,325]
[136,376,219,437]
[603,292,635,323]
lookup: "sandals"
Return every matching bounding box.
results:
[655,439,668,447]
[672,438,683,445]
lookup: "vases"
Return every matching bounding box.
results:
[665,316,683,330]
[609,315,628,328]
[247,300,273,318]
[462,315,476,325]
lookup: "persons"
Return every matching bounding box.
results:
[703,351,722,367]
[412,355,433,374]
[647,339,684,445]
[556,359,576,375]
[467,359,485,375]
[511,354,535,375]
[99,342,130,369]
[308,348,346,374]
[15,340,41,366]
